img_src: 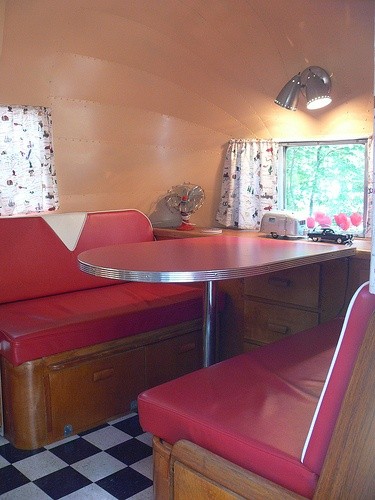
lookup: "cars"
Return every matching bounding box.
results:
[309,224,355,246]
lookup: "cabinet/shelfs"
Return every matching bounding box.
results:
[153,226,371,353]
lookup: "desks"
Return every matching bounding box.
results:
[77,236,356,368]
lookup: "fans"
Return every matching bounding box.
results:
[164,180,207,231]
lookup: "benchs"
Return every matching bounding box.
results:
[136,280,375,500]
[0,209,221,365]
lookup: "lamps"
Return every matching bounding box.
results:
[273,65,333,112]
